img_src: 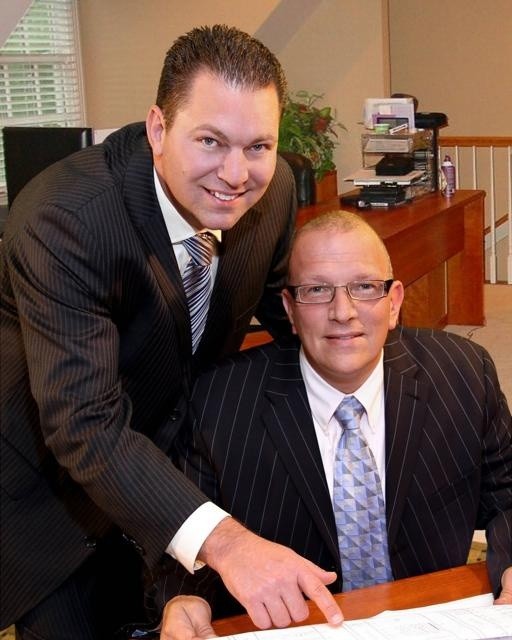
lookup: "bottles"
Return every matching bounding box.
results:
[439,154,457,198]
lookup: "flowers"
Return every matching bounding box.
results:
[277,89,348,185]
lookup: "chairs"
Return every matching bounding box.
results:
[275,151,313,206]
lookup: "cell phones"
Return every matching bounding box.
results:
[370,200,390,207]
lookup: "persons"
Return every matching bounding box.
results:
[0,23,346,640]
[141,208,511,640]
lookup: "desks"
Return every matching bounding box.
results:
[209,561,512,640]
[232,187,486,353]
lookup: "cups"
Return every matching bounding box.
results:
[374,123,390,135]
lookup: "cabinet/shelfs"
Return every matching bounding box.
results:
[358,123,440,203]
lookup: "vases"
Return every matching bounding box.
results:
[311,169,338,202]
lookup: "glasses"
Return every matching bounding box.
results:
[287,280,393,304]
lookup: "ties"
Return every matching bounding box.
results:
[181,233,216,356]
[332,397,395,593]
